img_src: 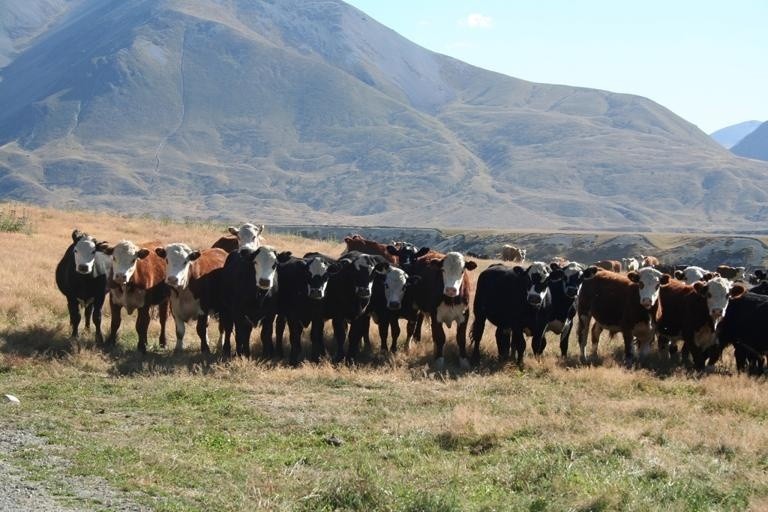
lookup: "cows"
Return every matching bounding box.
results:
[54,221,768,378]
